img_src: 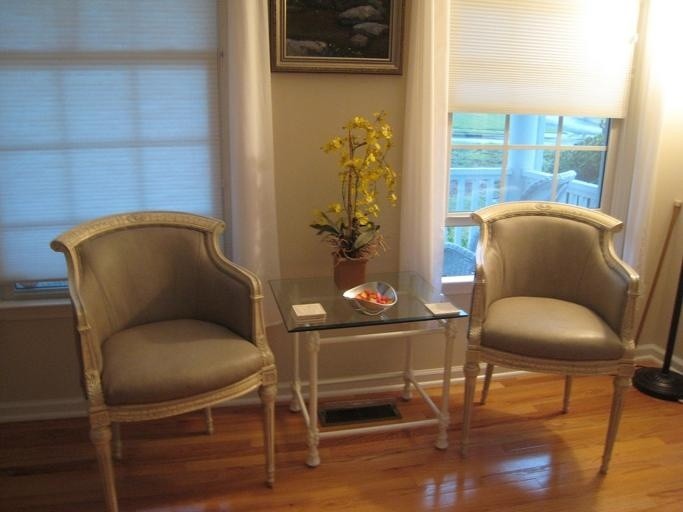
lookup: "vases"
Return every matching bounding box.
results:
[329,248,372,291]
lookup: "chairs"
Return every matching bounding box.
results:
[455,197,641,477]
[46,207,280,512]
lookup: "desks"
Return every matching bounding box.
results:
[264,266,474,473]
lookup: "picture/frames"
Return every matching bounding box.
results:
[268,0,408,79]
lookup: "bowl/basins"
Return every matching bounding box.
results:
[340,280,397,315]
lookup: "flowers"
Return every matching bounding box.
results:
[305,103,404,267]
